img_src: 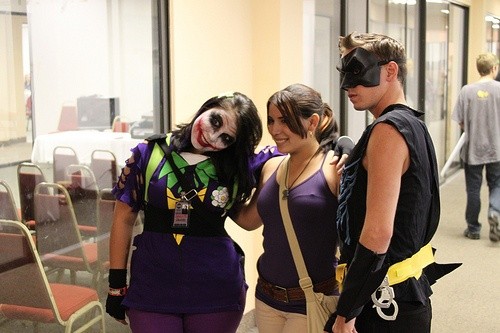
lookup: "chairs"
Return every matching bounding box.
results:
[112,116,127,132]
[0,145,145,333]
[57,102,77,131]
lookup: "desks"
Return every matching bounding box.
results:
[31,129,148,190]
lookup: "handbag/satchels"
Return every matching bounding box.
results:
[306,293,340,333]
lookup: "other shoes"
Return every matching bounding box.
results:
[488,212,500,243]
[463,228,480,239]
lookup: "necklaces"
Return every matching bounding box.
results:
[284,144,320,196]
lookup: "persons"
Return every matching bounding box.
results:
[227,83,346,333]
[104,92,355,333]
[451,51,500,242]
[331,30,462,333]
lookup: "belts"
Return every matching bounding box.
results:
[257,277,339,302]
